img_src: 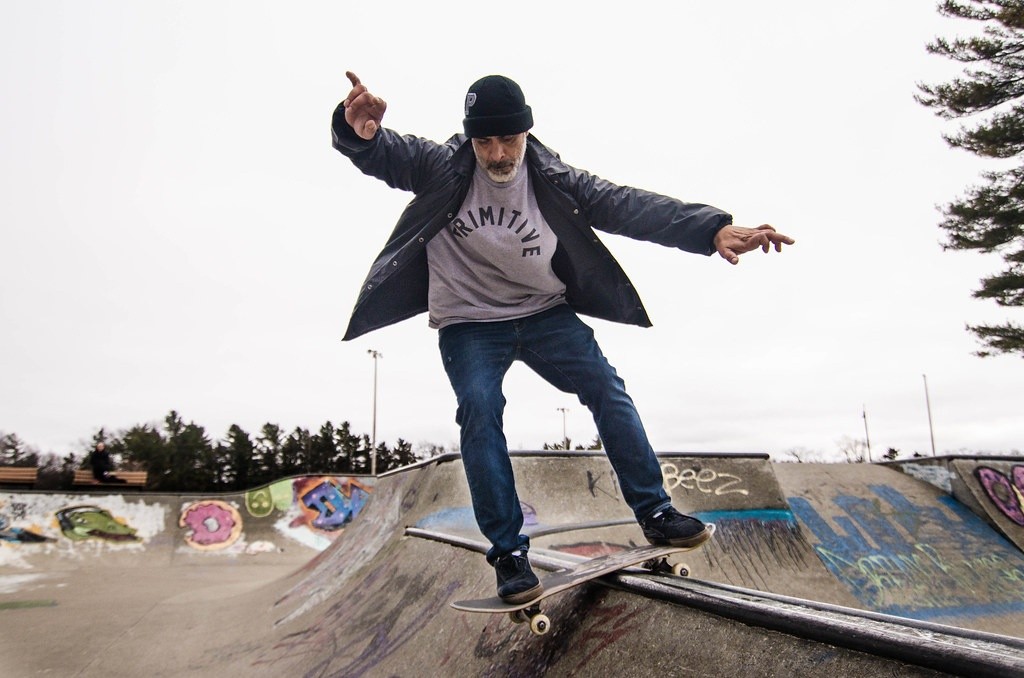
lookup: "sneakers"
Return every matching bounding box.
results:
[639,505,713,549]
[492,545,545,606]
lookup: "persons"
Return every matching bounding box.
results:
[332,71,795,606]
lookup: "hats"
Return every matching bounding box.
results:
[462,73,535,138]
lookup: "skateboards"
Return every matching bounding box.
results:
[449,521,717,636]
